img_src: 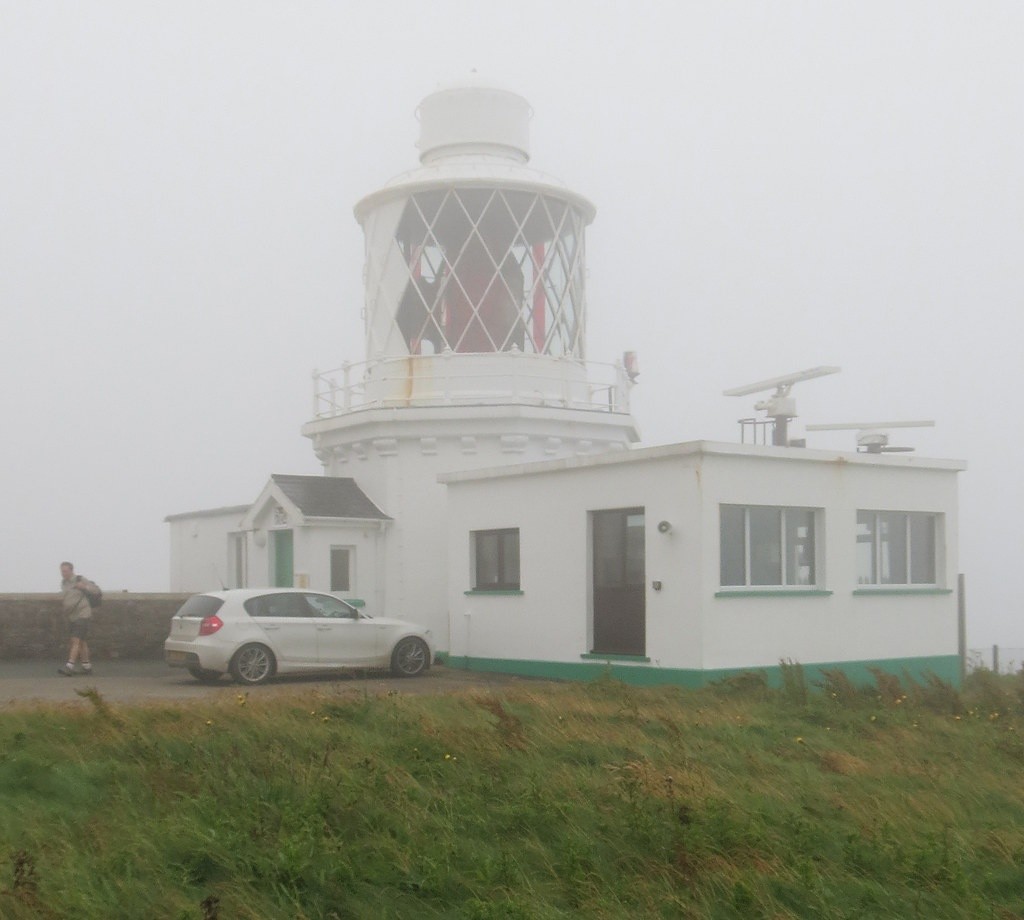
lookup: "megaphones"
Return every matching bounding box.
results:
[658,521,672,535]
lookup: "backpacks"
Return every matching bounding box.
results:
[76,575,102,608]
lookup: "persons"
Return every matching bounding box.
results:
[58,562,91,676]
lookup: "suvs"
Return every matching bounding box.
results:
[162,588,436,685]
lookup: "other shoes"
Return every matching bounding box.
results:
[57,666,73,676]
[74,666,91,674]
